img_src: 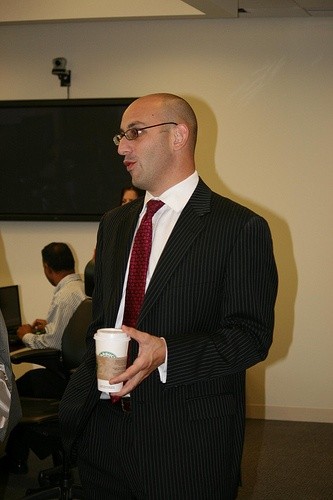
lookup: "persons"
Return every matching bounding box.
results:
[5,242,93,475]
[121,180,146,204]
[57,93,278,500]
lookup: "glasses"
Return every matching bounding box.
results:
[112,121,179,145]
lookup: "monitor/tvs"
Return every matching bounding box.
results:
[0,284,21,330]
[0,97,138,221]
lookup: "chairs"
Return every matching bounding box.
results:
[8,261,95,500]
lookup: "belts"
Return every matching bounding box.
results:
[105,397,132,413]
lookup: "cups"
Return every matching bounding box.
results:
[93,328,132,393]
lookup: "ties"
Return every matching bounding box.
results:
[122,199,165,386]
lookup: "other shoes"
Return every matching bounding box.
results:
[0,456,28,475]
